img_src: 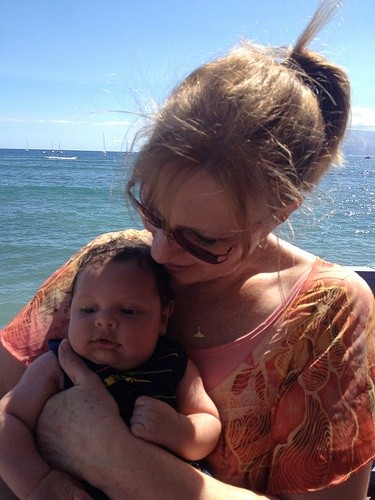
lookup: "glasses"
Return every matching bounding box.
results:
[124,173,260,262]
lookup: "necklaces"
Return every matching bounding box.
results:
[190,261,253,338]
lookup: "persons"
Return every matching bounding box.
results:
[0,249,222,500]
[0,0,375,500]
